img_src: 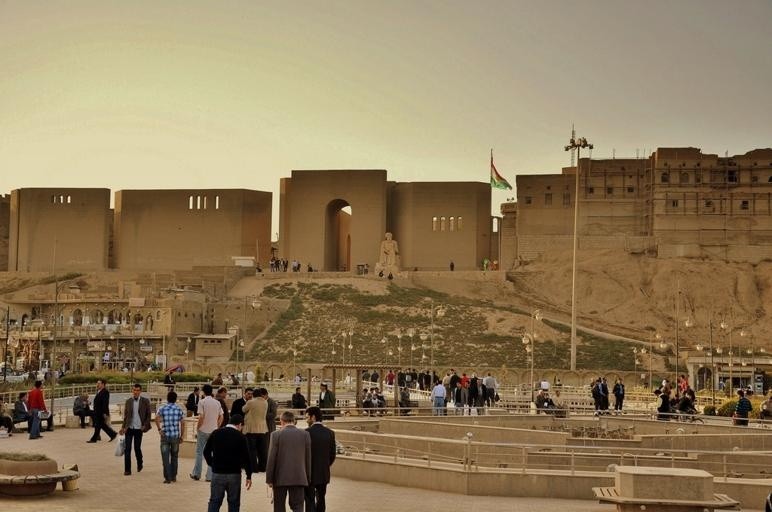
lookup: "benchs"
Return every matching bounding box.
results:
[273,400,308,418]
[336,399,420,414]
[0,467,82,496]
[152,402,158,416]
[5,409,29,434]
[530,402,570,418]
[66,414,111,427]
[185,399,195,417]
[590,482,742,512]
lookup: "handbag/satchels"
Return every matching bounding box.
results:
[37,410,52,420]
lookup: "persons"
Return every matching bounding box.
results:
[760,396,772,420]
[379,270,384,277]
[265,411,313,512]
[449,261,455,271]
[387,272,394,279]
[303,406,336,512]
[364,264,369,274]
[731,389,754,426]
[267,254,314,272]
[378,231,400,266]
[202,411,254,512]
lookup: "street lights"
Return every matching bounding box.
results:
[106,329,192,377]
[563,137,596,373]
[236,294,263,398]
[522,310,544,402]
[633,312,768,409]
[329,303,450,395]
[293,338,299,383]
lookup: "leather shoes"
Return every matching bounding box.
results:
[86,440,97,443]
[137,461,143,472]
[162,473,212,484]
[108,432,118,442]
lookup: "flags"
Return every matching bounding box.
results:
[490,156,513,192]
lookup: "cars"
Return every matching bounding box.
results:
[23,370,44,381]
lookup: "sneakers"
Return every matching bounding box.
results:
[124,471,131,476]
